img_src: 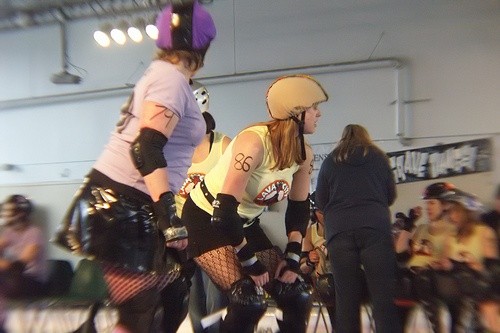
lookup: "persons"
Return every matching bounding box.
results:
[301,209,335,321]
[0,195,49,333]
[315,124,401,333]
[182,75,328,333]
[173,80,233,333]
[51,0,216,333]
[392,182,500,333]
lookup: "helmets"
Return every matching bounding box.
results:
[8,194,32,214]
[266,73,328,119]
[310,190,320,210]
[422,181,457,203]
[190,80,209,112]
[155,0,216,49]
[446,192,482,210]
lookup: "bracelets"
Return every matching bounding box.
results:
[305,259,313,266]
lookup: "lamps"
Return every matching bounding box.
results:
[93,8,159,48]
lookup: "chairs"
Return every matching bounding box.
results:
[0,260,118,333]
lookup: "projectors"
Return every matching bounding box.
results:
[50,71,82,84]
